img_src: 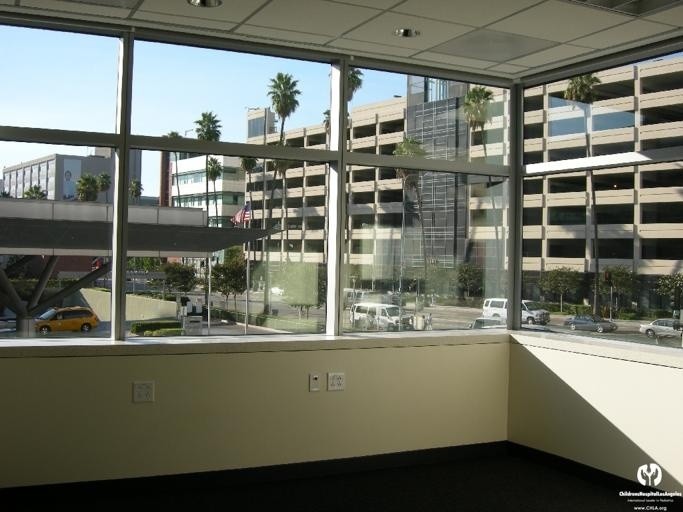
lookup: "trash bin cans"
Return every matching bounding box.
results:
[272,309,279,316]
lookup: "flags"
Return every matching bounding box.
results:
[228,203,251,225]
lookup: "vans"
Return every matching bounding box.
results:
[481,297,550,326]
[350,303,413,331]
[342,288,384,310]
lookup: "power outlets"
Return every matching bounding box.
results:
[327,372,345,390]
[132,382,154,404]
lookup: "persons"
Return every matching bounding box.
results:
[425,313,432,331]
[420,315,426,329]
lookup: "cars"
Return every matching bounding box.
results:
[568,314,618,333]
[271,287,285,296]
[639,318,682,340]
[33,305,98,336]
